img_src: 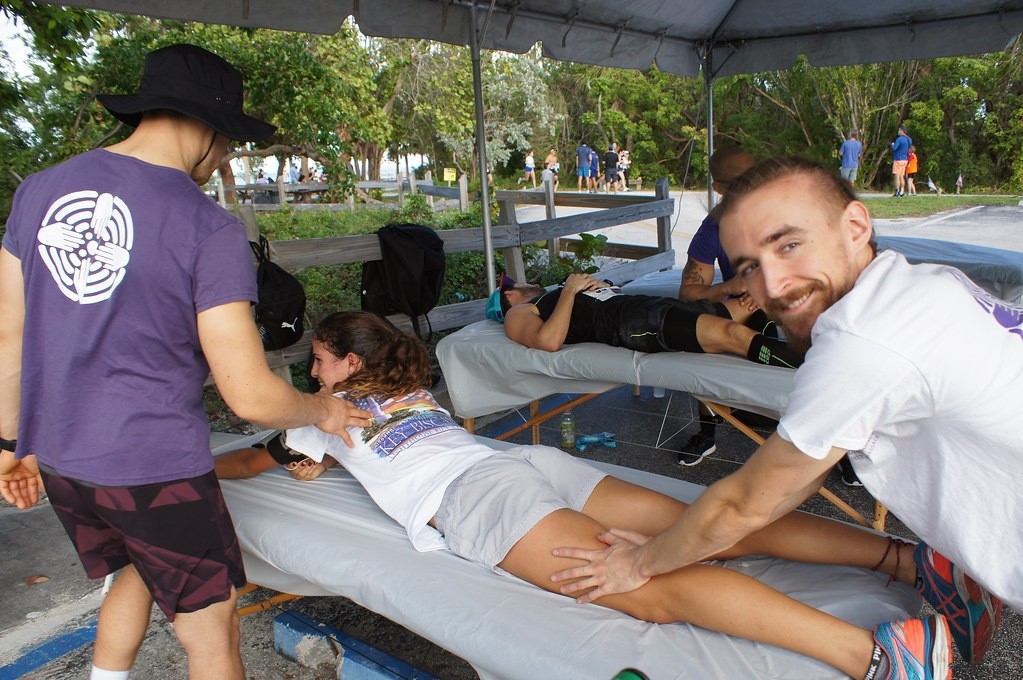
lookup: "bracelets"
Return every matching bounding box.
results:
[0,437,17,452]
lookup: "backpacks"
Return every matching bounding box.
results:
[249,233,306,352]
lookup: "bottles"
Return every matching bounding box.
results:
[653,386,664,397]
[560,410,575,449]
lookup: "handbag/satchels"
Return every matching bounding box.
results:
[907,150,914,165]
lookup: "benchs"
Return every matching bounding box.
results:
[598,178,642,190]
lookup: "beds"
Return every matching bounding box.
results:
[212,236,1023,680]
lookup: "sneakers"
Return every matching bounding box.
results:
[837,454,863,486]
[911,540,1001,665]
[677,434,716,466]
[874,612,954,680]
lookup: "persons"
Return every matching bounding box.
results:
[517,138,632,194]
[256,162,329,201]
[210,309,1004,680]
[675,144,867,487]
[550,152,1023,617]
[890,124,912,198]
[487,160,492,185]
[905,145,918,196]
[0,43,373,678]
[838,126,863,189]
[483,268,807,370]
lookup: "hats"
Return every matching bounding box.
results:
[95,44,277,142]
[608,146,613,149]
[485,272,514,324]
[900,126,908,134]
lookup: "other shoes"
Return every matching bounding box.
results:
[628,188,632,191]
[603,185,606,191]
[623,187,630,192]
[518,177,521,184]
[893,189,905,197]
[579,190,598,194]
